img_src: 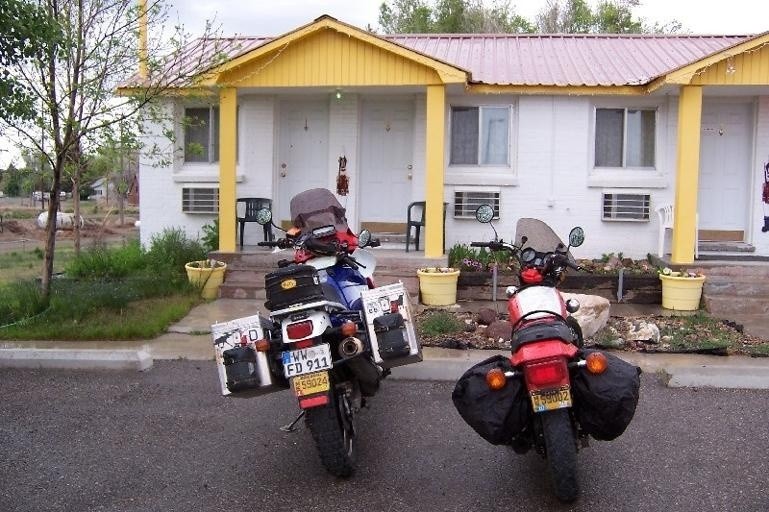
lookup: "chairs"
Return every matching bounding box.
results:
[235,198,272,249]
[406,201,450,254]
[654,200,699,259]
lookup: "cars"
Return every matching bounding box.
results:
[32,190,73,203]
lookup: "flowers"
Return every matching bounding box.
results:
[458,257,495,273]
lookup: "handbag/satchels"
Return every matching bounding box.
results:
[452,355,532,445]
[570,349,642,441]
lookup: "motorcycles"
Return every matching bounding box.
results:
[252,185,381,477]
[466,203,592,508]
